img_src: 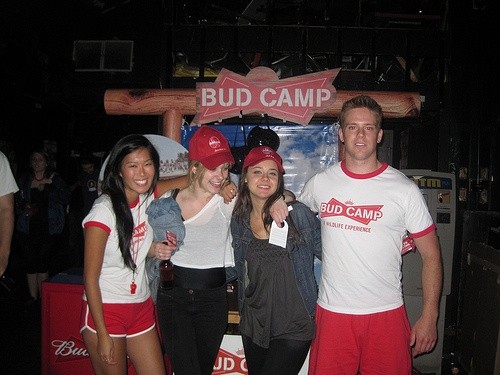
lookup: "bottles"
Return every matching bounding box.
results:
[160,241,174,289]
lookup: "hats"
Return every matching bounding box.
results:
[189,125,235,170]
[242,145,285,174]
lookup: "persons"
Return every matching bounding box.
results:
[224,145,323,375]
[296,95,443,375]
[80,134,239,375]
[157,127,296,375]
[0,150,112,309]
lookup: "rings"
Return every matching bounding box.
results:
[230,188,234,194]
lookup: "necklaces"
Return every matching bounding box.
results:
[34,176,45,182]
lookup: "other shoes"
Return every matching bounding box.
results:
[30,296,41,307]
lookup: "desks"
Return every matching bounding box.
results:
[40,267,172,375]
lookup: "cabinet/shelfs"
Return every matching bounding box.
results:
[458,239,500,375]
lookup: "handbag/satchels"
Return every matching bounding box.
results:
[247,115,281,152]
